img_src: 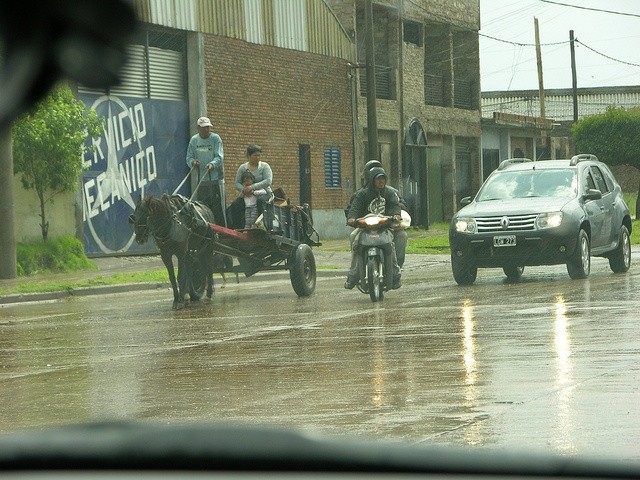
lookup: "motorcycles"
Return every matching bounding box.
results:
[346,213,403,301]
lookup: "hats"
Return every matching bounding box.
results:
[376,173,384,178]
[198,117,213,127]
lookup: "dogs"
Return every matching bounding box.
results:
[212,252,239,289]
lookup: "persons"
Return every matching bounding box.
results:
[345,159,408,269]
[240,174,266,229]
[186,118,229,227]
[231,143,275,230]
[344,167,404,290]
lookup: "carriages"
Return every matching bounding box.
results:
[129,193,322,311]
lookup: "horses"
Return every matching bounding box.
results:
[133,195,215,311]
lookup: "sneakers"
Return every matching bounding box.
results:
[344,279,356,289]
[393,280,402,289]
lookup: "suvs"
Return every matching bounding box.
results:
[449,154,632,286]
